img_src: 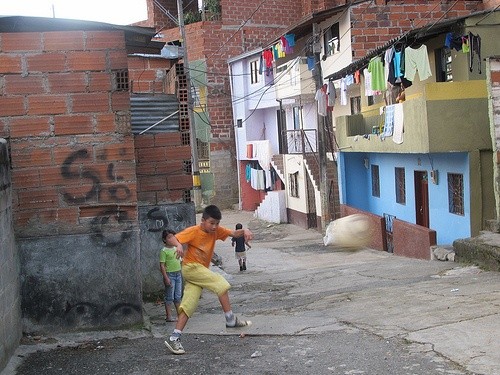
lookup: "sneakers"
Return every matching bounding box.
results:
[226,317,252,330]
[164,335,185,354]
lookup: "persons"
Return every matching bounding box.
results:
[231,223,252,271]
[159,228,182,323]
[164,205,253,355]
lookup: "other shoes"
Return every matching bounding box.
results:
[243,262,246,270]
[166,317,177,322]
[240,266,243,271]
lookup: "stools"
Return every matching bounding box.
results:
[372,126,384,134]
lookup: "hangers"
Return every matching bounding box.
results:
[394,38,402,49]
[409,36,422,47]
